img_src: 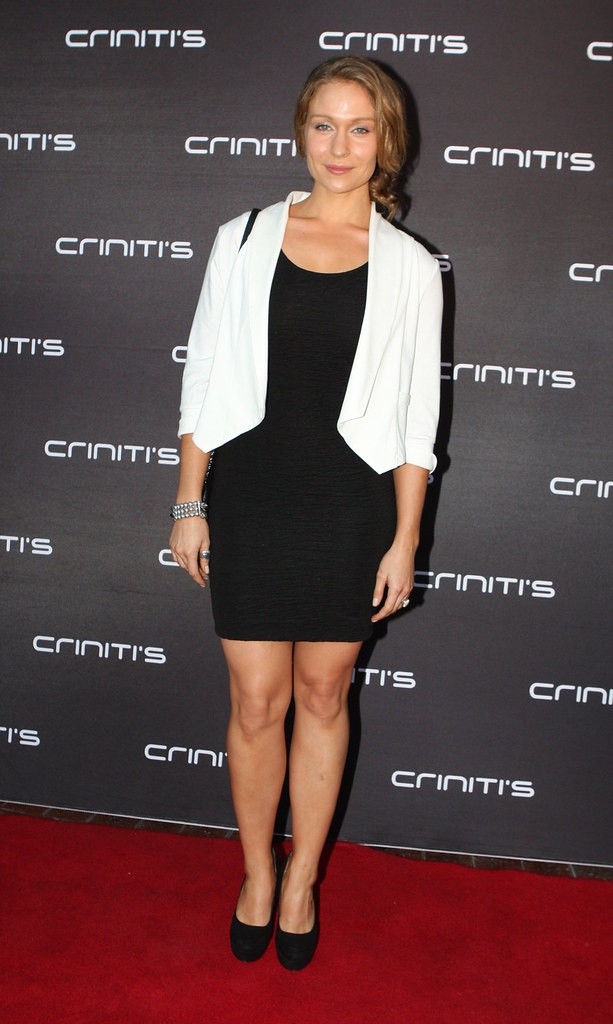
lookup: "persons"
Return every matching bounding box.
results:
[168,53,444,972]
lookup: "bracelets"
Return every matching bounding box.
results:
[170,501,209,522]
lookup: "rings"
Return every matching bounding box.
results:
[199,550,211,559]
[401,599,410,608]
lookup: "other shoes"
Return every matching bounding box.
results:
[230,848,279,962]
[276,853,320,970]
